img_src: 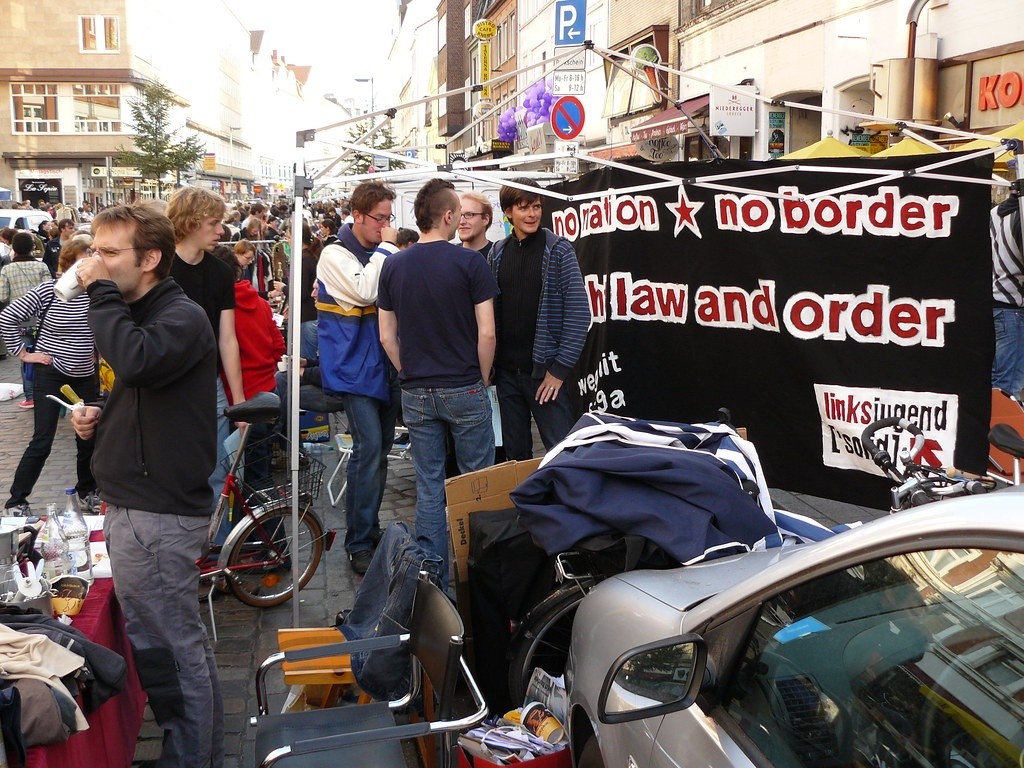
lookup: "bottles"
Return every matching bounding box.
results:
[60,486,95,586]
[303,442,334,454]
[41,503,76,580]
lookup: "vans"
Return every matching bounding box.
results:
[0,207,54,257]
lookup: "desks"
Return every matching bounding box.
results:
[26,517,148,768]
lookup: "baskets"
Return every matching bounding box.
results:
[220,433,328,519]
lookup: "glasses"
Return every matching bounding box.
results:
[357,210,396,224]
[460,211,489,220]
[241,253,254,263]
[86,246,153,261]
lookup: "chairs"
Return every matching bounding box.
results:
[250,570,489,767]
[327,426,414,507]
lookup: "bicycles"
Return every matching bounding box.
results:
[504,414,990,708]
[43,383,339,642]
[986,422,1024,487]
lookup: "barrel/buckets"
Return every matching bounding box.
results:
[0,579,53,617]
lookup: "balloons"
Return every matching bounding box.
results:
[497,105,523,142]
[524,78,561,128]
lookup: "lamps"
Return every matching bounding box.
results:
[943,112,966,129]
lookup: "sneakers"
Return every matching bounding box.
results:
[370,531,385,550]
[75,493,101,514]
[348,550,372,574]
[19,398,36,409]
[2,499,33,519]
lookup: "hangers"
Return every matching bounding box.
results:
[256,242,272,254]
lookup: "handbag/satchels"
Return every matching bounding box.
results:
[22,345,35,380]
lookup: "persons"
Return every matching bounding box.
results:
[990,193,1024,399]
[376,179,501,593]
[455,193,506,465]
[487,177,591,461]
[314,181,403,573]
[71,206,223,768]
[0,187,419,600]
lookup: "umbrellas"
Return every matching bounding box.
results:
[776,118,1024,172]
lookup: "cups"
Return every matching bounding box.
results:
[519,701,565,745]
[53,257,85,304]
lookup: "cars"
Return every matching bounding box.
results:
[561,485,1024,768]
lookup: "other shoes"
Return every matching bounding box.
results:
[238,556,292,574]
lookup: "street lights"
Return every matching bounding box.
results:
[228,126,243,204]
[353,77,375,173]
